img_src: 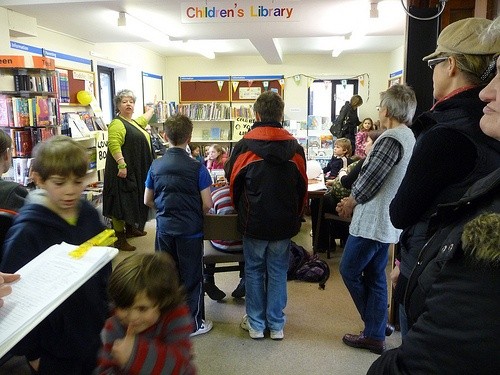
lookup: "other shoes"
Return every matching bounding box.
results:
[232,276,246,297]
[203,274,226,300]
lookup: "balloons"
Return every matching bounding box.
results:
[77,91,91,105]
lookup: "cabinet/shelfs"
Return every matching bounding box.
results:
[0,54,107,214]
[180,100,255,153]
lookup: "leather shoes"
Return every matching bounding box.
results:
[385,323,395,336]
[343,331,386,355]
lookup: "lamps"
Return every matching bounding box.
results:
[331,34,349,58]
[118,12,170,43]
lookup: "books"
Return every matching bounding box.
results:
[61,111,108,138]
[158,100,230,120]
[0,76,61,192]
[231,105,256,119]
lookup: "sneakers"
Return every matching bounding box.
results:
[241,314,264,339]
[189,320,213,337]
[270,329,283,339]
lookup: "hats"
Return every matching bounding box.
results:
[423,17,500,61]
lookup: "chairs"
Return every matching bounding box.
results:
[324,211,351,258]
[204,212,244,273]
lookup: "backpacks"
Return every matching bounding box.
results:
[288,240,330,289]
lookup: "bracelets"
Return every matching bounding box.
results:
[116,157,124,162]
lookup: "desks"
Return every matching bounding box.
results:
[306,169,326,254]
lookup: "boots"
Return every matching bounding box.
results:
[114,231,136,251]
[126,225,147,238]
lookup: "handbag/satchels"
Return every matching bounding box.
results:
[329,120,349,139]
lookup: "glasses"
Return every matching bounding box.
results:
[427,56,449,68]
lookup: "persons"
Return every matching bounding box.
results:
[102,89,158,251]
[189,142,229,169]
[309,117,385,254]
[0,272,21,307]
[365,15,499,375]
[340,95,363,155]
[336,83,418,355]
[224,90,308,339]
[389,20,500,342]
[0,129,28,251]
[97,251,198,375]
[144,112,213,337]
[201,159,245,300]
[2,134,112,375]
